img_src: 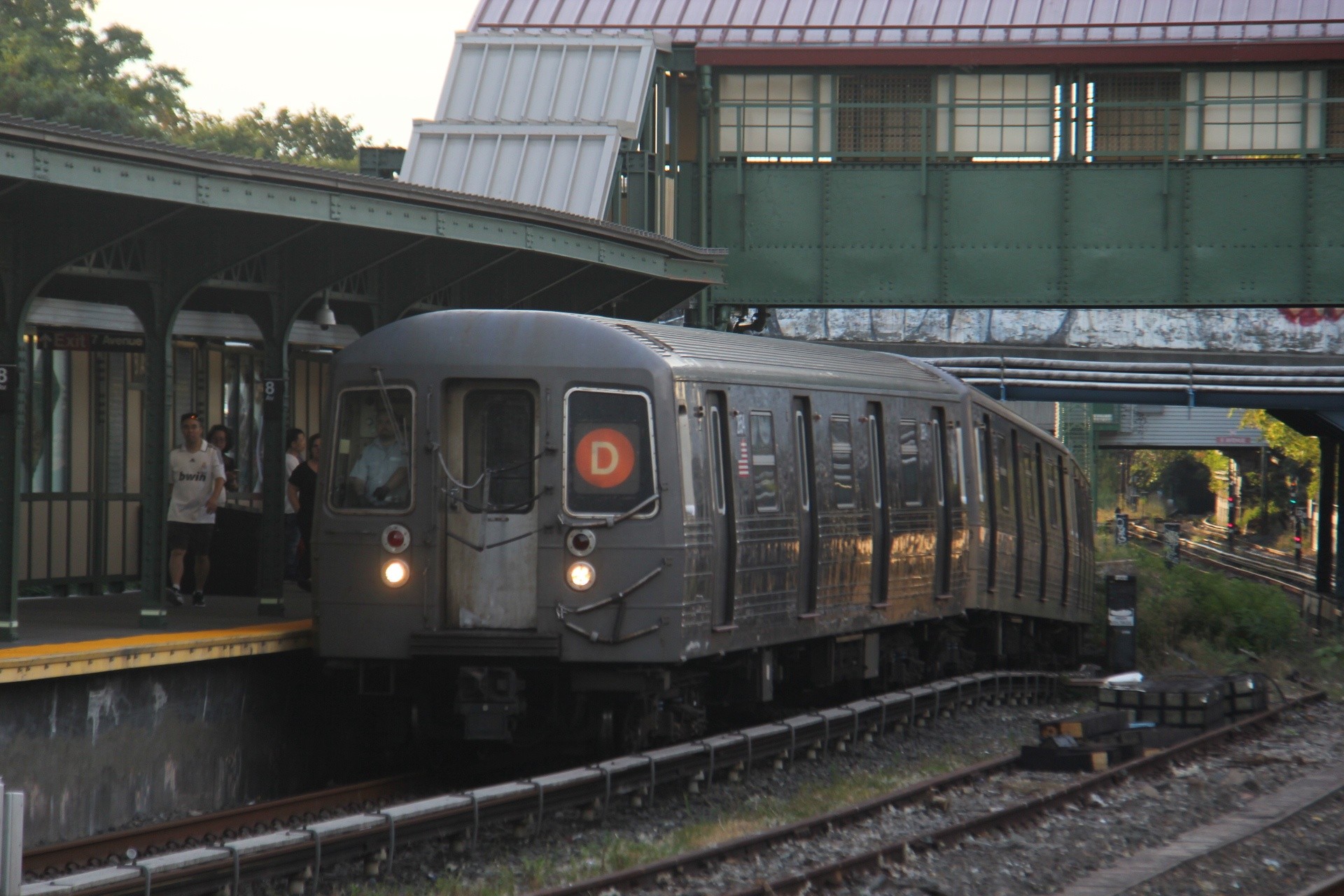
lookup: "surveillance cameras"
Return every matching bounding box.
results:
[316,309,337,330]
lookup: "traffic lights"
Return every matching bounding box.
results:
[1227,507,1236,529]
[1289,476,1298,504]
[1294,518,1302,544]
[1227,484,1235,504]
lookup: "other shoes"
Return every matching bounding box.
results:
[164,586,185,606]
[191,592,207,609]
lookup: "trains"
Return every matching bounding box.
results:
[307,307,1101,749]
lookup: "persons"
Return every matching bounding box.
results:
[166,413,240,607]
[284,428,322,592]
[349,408,410,508]
